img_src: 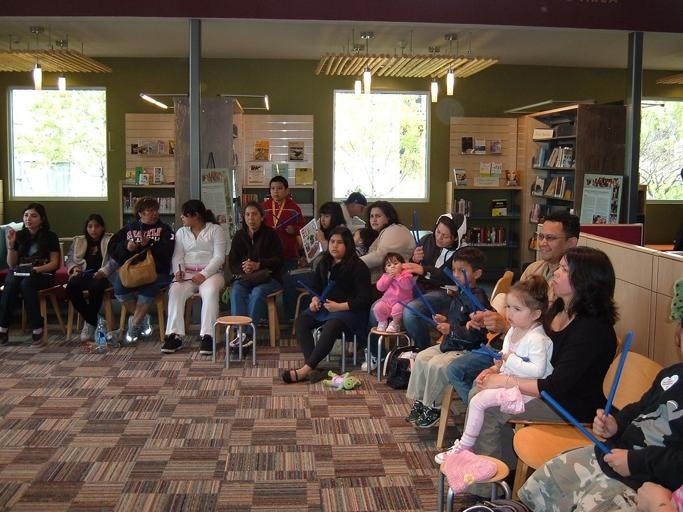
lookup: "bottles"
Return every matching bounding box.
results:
[95,318,106,346]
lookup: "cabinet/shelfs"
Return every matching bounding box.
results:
[446,181,523,285]
[242,180,318,226]
[119,179,175,234]
[172,95,244,284]
[523,102,626,277]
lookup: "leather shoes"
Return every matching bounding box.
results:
[281,368,305,383]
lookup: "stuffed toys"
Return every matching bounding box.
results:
[324,369,361,390]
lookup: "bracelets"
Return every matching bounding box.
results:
[7,248,14,250]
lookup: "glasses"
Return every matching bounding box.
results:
[538,234,569,241]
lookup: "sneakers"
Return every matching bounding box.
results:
[435,439,473,463]
[0,330,8,345]
[233,336,252,350]
[199,334,212,354]
[139,313,153,336]
[405,400,424,422]
[414,404,441,428]
[29,326,44,347]
[376,320,388,332]
[224,333,246,350]
[160,332,182,353]
[361,354,385,372]
[125,315,139,345]
[386,321,400,332]
[80,322,92,342]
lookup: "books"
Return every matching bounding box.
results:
[120,194,176,234]
[297,205,314,217]
[453,135,573,247]
[247,140,313,186]
[242,193,258,209]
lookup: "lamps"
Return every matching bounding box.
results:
[445,33,458,96]
[429,46,440,102]
[217,94,270,111]
[30,26,45,90]
[56,39,68,98]
[361,32,376,95]
[139,93,188,109]
[353,43,364,99]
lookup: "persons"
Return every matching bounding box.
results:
[161,200,226,355]
[404,214,618,511]
[65,213,110,342]
[261,176,305,341]
[108,197,175,346]
[281,191,465,384]
[229,201,286,349]
[0,203,61,346]
[514,308,683,512]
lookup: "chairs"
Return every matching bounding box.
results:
[226,287,283,346]
[113,286,168,342]
[506,336,623,434]
[292,286,311,336]
[436,293,507,449]
[512,351,664,500]
[436,271,513,345]
[62,284,114,341]
[185,288,224,343]
[0,242,66,343]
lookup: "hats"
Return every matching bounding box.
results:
[444,448,497,494]
[341,192,367,206]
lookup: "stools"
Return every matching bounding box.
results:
[313,325,357,372]
[437,455,511,511]
[212,316,256,369]
[367,327,411,382]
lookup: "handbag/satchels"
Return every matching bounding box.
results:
[387,345,423,389]
[8,257,48,278]
[118,256,157,288]
[233,268,272,286]
